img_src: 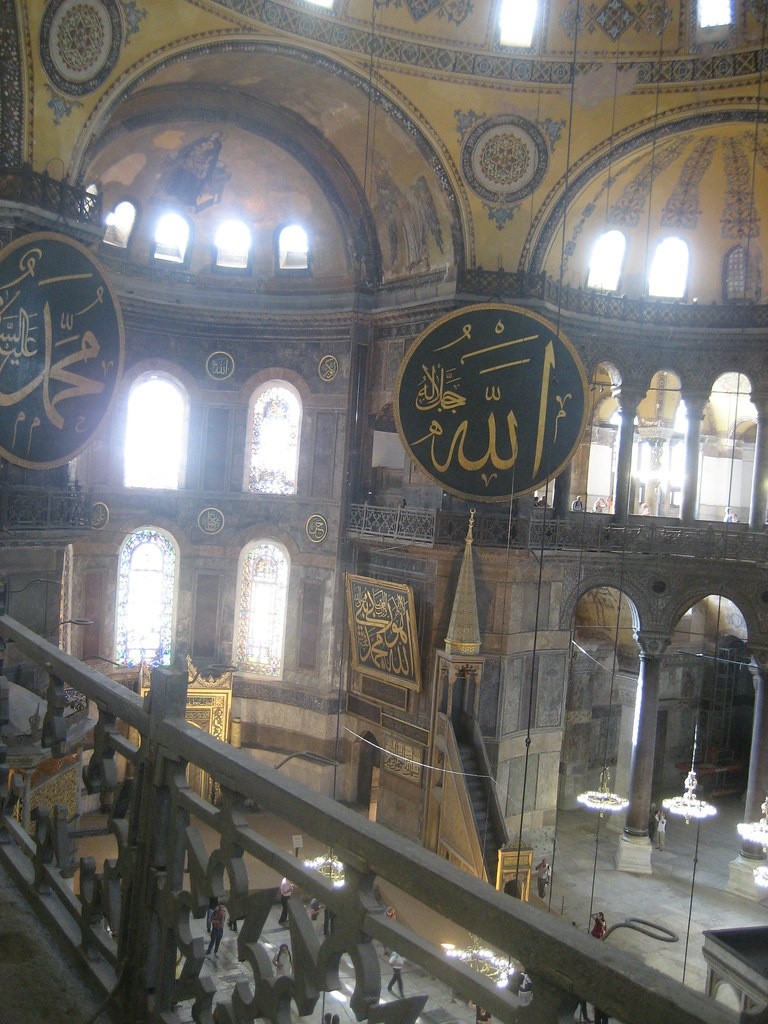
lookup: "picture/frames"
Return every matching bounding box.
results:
[344,572,424,695]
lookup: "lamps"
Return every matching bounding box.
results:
[577,761,632,812]
[446,932,515,987]
[661,772,718,825]
[302,845,345,888]
[736,796,768,889]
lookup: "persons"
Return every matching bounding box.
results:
[723,508,740,522]
[272,944,292,977]
[324,905,335,936]
[279,878,293,923]
[592,912,606,938]
[206,904,237,958]
[311,897,323,928]
[649,803,666,851]
[388,950,405,999]
[592,1005,611,1024]
[594,496,610,513]
[517,971,532,1002]
[572,497,584,511]
[534,496,545,507]
[642,504,650,515]
[536,859,551,899]
[470,1004,492,1024]
[384,907,397,955]
